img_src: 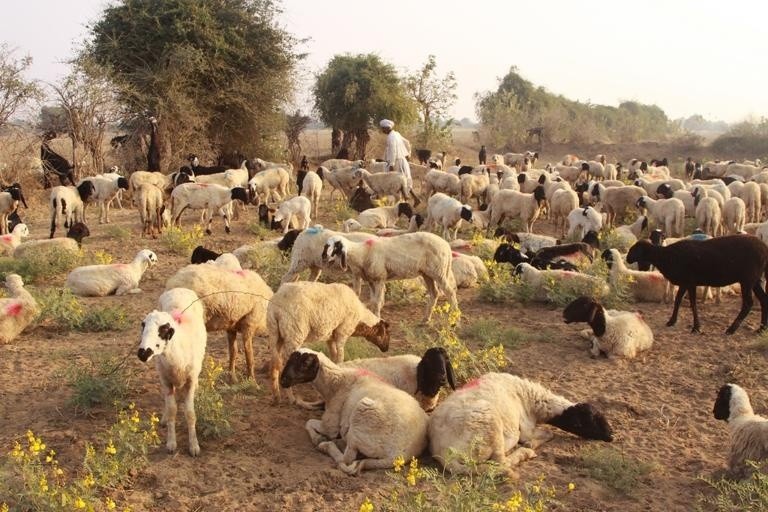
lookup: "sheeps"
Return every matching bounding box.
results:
[40,130,76,189]
[525,126,545,144]
[0,273,41,344]
[50,146,768,477]
[13,222,90,262]
[147,112,160,172]
[0,183,30,258]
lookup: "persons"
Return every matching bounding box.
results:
[478,145,487,165]
[685,157,696,182]
[693,162,702,179]
[191,156,201,172]
[109,166,115,173]
[379,119,422,208]
[113,165,120,173]
[300,154,309,170]
